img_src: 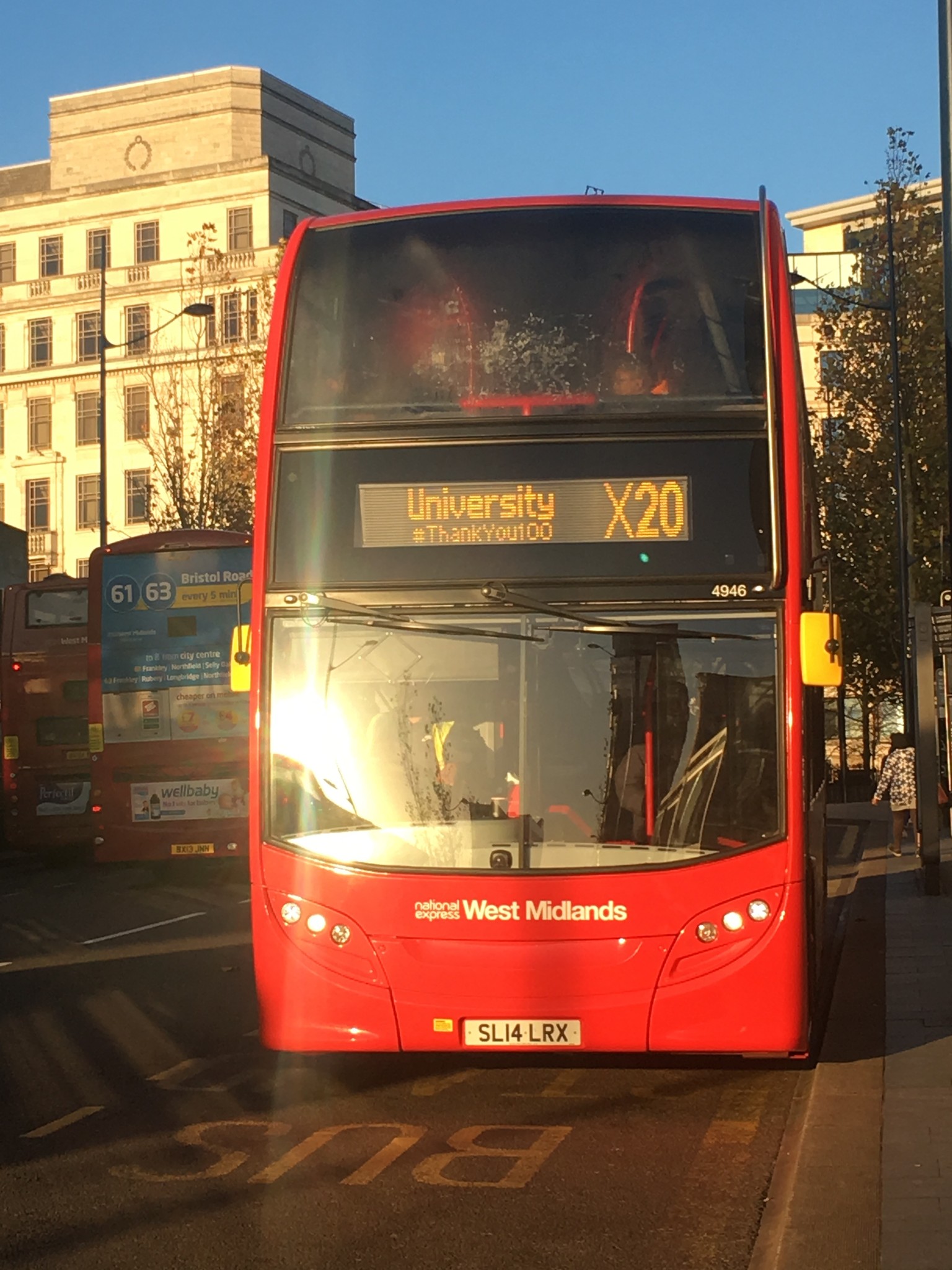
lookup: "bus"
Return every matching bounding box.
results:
[88,531,253,865]
[230,194,840,1062]
[0,574,90,851]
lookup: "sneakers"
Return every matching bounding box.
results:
[886,843,902,857]
[915,848,920,858]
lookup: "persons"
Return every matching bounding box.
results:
[142,801,148,812]
[651,327,750,395]
[872,733,920,857]
[440,721,493,788]
[367,712,451,820]
[613,361,650,395]
[614,743,646,841]
[938,719,947,777]
[230,778,248,810]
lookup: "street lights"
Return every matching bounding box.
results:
[99,235,215,544]
[789,194,911,734]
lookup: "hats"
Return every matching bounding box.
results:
[891,733,908,748]
